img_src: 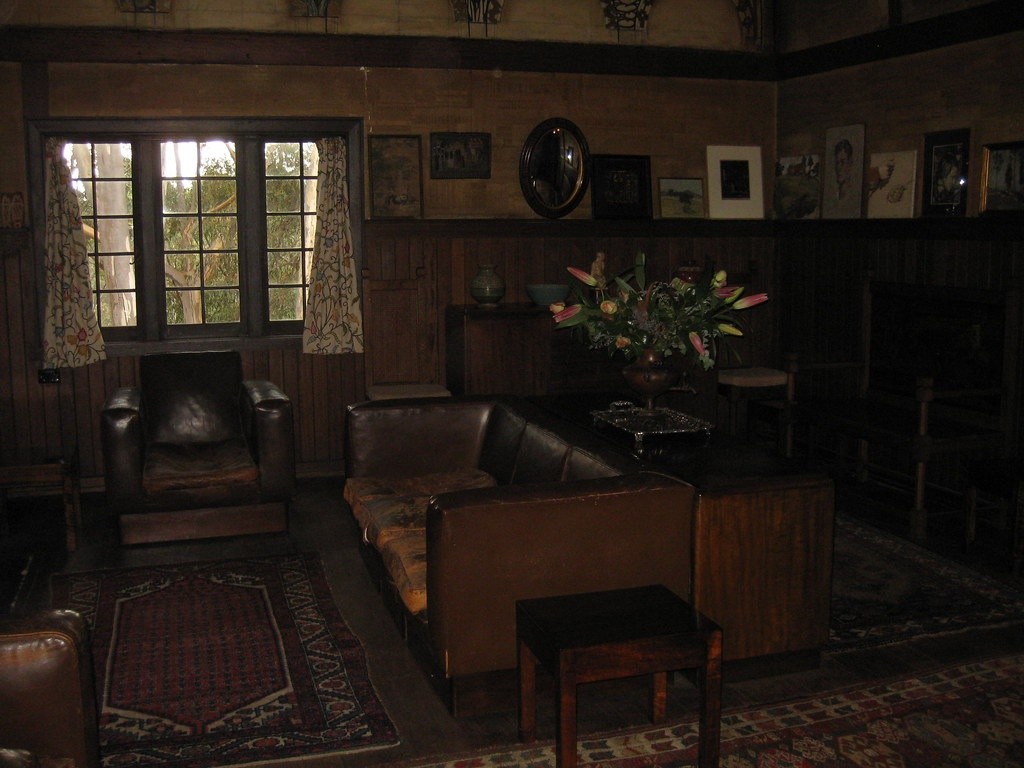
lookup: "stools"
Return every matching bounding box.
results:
[0,446,85,552]
[365,383,450,403]
[516,582,723,768]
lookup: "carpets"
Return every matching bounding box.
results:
[370,652,1024,768]
[47,551,401,768]
[821,514,1024,656]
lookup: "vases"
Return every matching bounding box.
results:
[621,345,678,420]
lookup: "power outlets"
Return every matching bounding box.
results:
[39,368,60,383]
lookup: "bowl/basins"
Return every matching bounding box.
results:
[525,284,570,307]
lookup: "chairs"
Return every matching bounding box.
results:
[713,314,792,439]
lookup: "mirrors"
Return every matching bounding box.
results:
[519,118,593,219]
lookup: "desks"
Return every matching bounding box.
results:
[445,302,551,394]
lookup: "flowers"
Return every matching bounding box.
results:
[547,254,768,372]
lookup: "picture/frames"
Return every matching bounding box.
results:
[366,131,425,220]
[590,120,1024,218]
[428,132,493,179]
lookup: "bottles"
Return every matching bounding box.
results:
[470,263,503,308]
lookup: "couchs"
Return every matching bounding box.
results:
[99,351,294,542]
[1,605,101,768]
[341,392,697,719]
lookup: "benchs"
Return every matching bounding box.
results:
[783,277,1023,545]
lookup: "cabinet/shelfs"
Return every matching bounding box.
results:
[541,391,839,661]
